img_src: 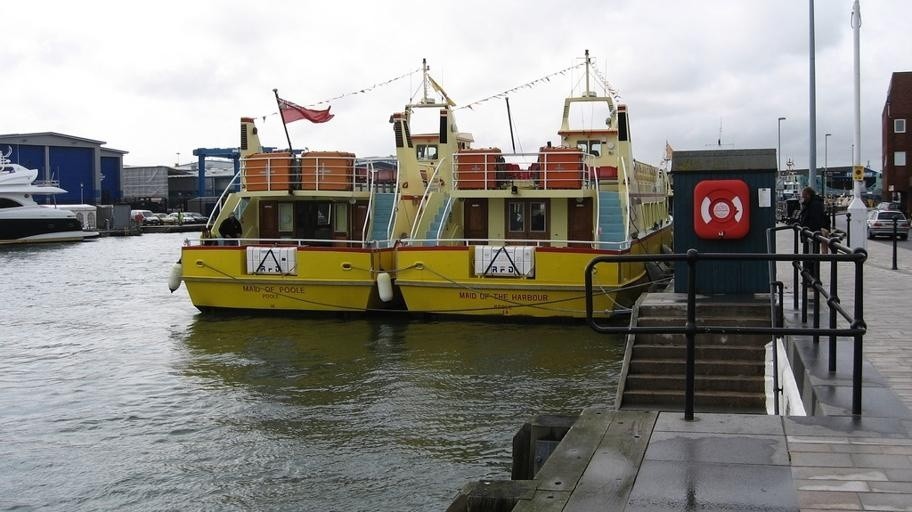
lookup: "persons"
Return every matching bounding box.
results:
[200,226,219,245]
[787,185,828,288]
[218,212,244,246]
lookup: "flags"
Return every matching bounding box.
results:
[665,142,673,161]
[426,74,457,108]
[276,96,335,126]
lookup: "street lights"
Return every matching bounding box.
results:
[824,133,831,196]
[777,117,786,177]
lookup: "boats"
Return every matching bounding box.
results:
[0,146,100,246]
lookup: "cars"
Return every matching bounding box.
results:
[865,207,908,242]
[129,209,210,226]
